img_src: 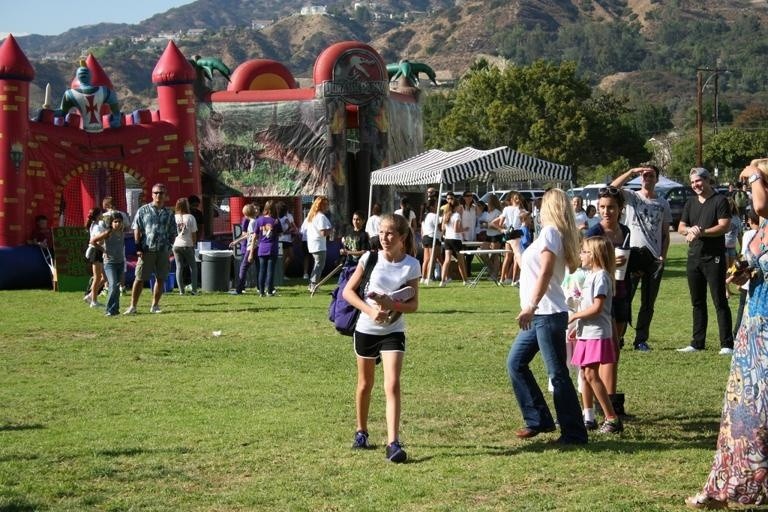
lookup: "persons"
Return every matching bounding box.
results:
[606,165,672,349]
[546,265,599,430]
[677,168,759,355]
[32,213,57,290]
[85,182,211,315]
[366,187,597,286]
[229,196,334,298]
[566,234,625,434]
[682,159,768,510]
[587,186,639,421]
[340,212,422,464]
[504,186,589,446]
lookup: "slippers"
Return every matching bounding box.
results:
[684,492,723,509]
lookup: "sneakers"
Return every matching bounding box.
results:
[389,284,416,302]
[584,419,598,431]
[84,295,105,307]
[389,309,402,324]
[420,275,520,288]
[386,440,406,462]
[600,417,623,432]
[677,345,701,352]
[354,431,369,447]
[123,307,136,315]
[228,289,276,297]
[635,342,651,350]
[719,347,733,355]
[150,306,161,313]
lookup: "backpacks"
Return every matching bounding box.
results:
[328,249,378,336]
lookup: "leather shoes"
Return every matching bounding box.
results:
[516,427,556,437]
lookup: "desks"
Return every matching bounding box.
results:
[440,240,513,290]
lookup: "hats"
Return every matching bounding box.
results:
[688,168,715,185]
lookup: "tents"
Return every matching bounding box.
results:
[367,146,574,284]
[627,174,682,233]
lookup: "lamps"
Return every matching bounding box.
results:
[10,140,25,174]
[183,139,195,170]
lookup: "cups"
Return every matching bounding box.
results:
[614,245,631,281]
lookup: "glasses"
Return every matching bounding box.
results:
[154,192,164,194]
[581,248,592,254]
[599,188,620,194]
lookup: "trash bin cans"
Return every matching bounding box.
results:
[198,249,232,292]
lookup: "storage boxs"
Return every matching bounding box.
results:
[149,271,176,292]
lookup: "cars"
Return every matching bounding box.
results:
[432,183,729,229]
